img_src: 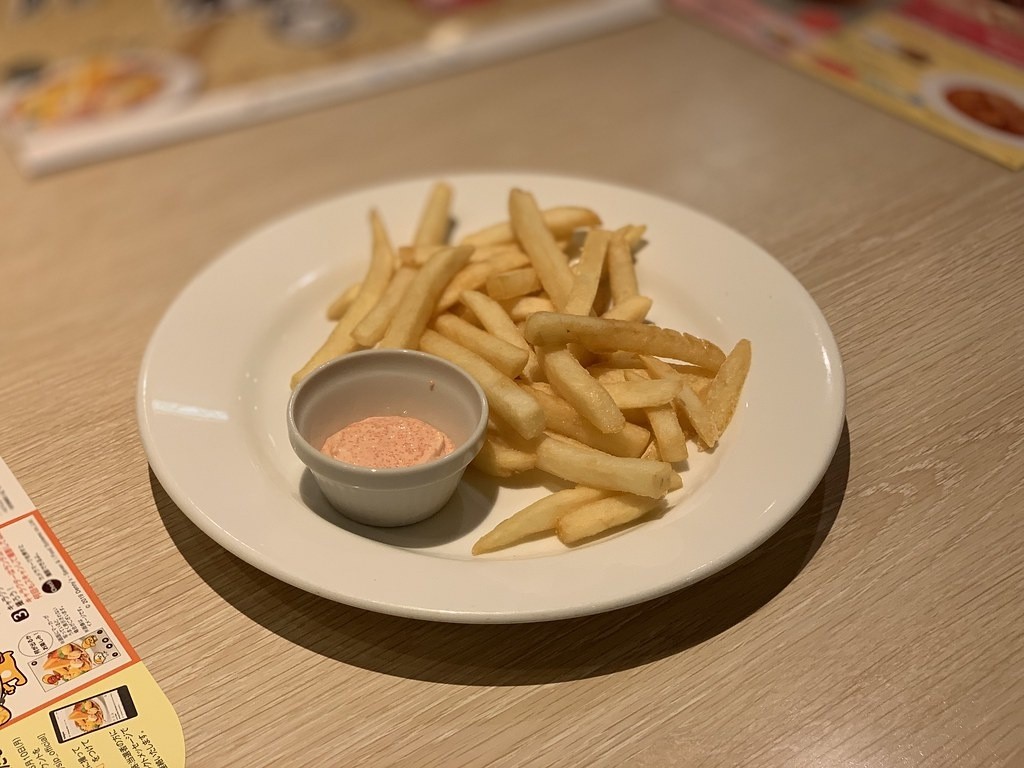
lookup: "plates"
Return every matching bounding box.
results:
[135,173,848,622]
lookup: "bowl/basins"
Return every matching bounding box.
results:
[289,346,488,528]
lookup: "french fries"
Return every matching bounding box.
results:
[288,179,751,558]
